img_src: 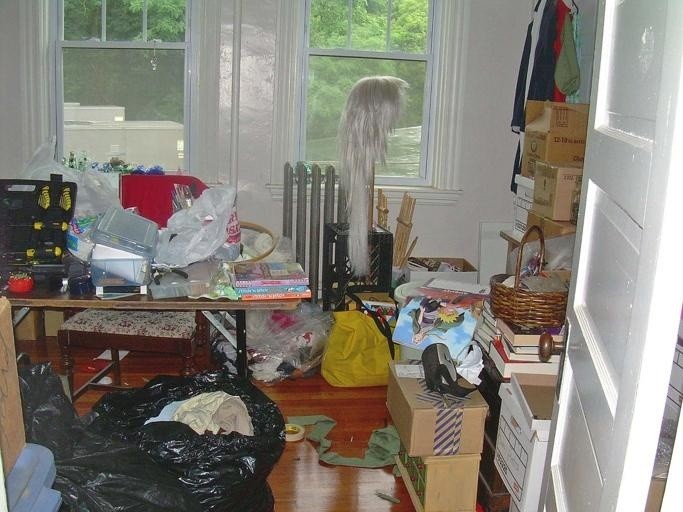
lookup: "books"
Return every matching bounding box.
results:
[240,287,312,302]
[471,298,568,380]
[234,261,311,287]
[226,271,307,294]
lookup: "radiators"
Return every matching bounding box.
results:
[281,161,347,308]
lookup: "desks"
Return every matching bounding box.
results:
[1,236,300,380]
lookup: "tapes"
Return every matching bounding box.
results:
[285,423,305,442]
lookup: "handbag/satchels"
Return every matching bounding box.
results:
[319,282,399,388]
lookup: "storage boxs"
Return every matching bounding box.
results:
[343,97,590,512]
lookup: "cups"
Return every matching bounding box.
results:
[67,274,90,294]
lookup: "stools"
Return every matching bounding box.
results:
[57,309,200,404]
